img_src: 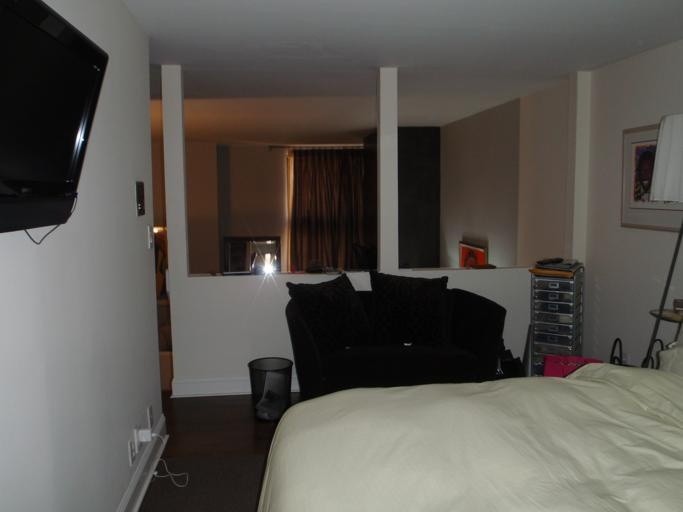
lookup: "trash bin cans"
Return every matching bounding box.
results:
[247,357,293,422]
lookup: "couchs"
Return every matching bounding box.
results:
[283,290,506,383]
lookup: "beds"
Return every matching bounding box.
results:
[257,339,682,510]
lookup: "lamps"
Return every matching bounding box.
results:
[634,114,682,370]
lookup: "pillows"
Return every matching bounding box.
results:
[372,268,449,342]
[289,275,365,346]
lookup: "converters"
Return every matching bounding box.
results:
[139,428,151,442]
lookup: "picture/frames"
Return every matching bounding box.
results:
[616,127,683,231]
[457,239,487,269]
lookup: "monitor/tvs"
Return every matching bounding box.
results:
[0,0,109,233]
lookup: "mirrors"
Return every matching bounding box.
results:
[222,237,280,273]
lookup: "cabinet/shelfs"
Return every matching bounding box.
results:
[531,267,582,377]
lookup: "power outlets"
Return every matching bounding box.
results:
[133,427,142,452]
[145,404,155,428]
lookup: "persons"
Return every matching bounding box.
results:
[635,151,653,200]
